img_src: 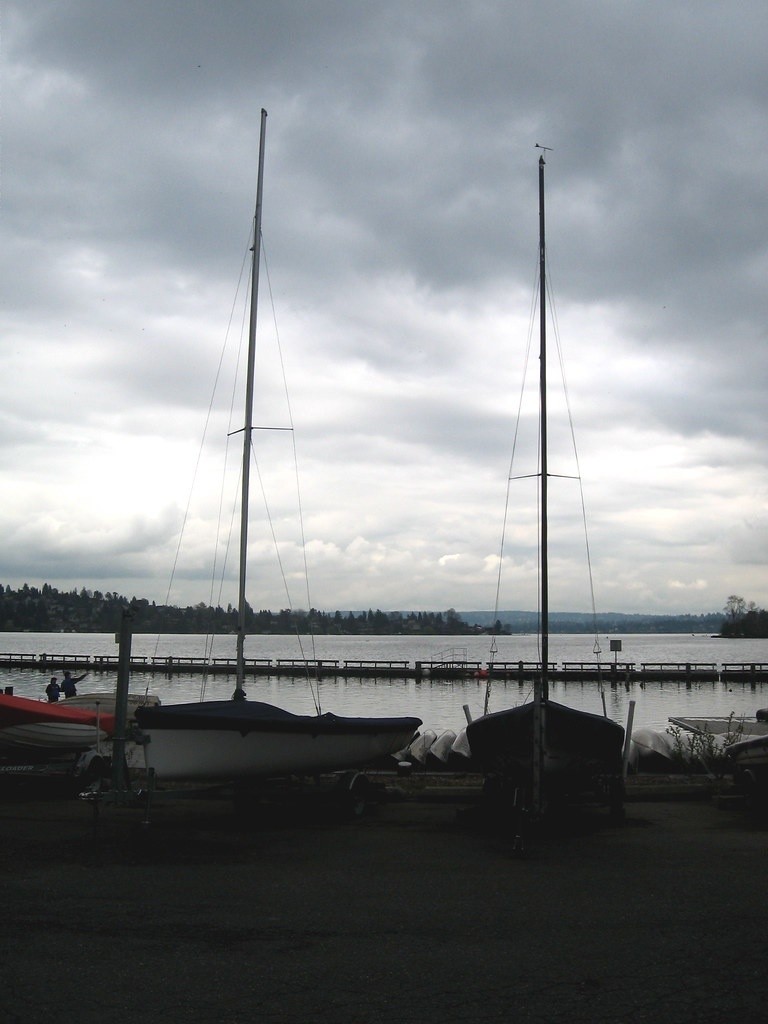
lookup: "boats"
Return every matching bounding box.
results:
[0,693,161,758]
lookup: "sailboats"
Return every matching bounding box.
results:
[466,144,624,824]
[138,109,422,786]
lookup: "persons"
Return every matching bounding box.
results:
[61,672,92,698]
[46,678,60,702]
